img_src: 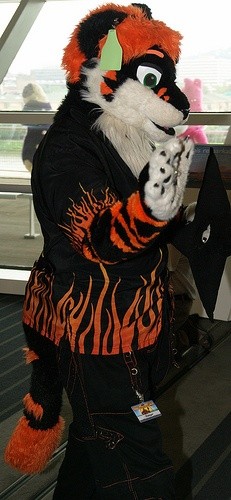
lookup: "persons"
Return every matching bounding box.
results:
[21,83,54,173]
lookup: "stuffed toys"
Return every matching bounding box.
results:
[177,78,208,143]
[3,2,199,500]
